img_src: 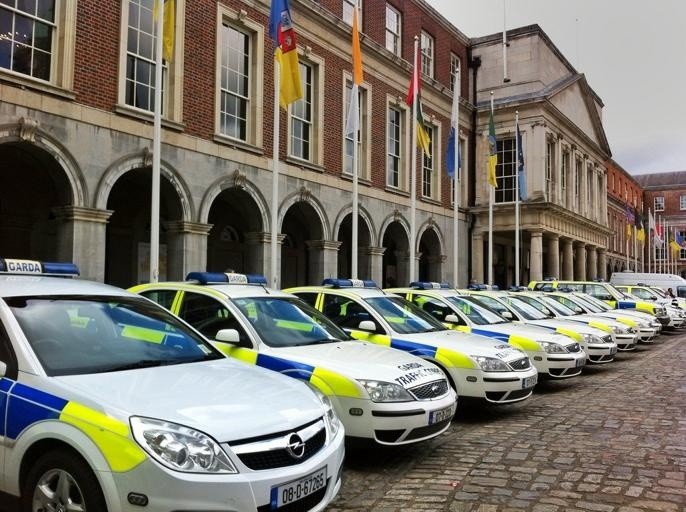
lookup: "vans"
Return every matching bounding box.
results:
[608,270,686,313]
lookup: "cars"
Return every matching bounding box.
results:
[103,271,459,449]
[217,279,540,410]
[0,258,347,511]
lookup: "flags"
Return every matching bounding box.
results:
[517,123,528,200]
[406,46,431,159]
[488,106,499,187]
[346,6,365,135]
[445,80,462,178]
[154,1,175,64]
[624,198,686,260]
[268,0,303,112]
